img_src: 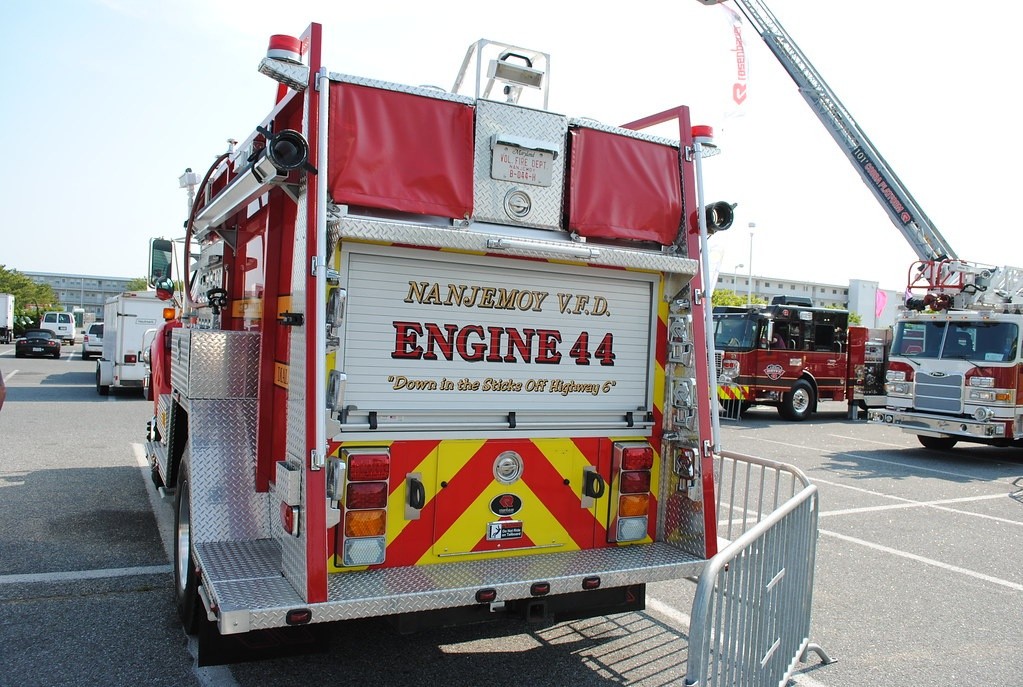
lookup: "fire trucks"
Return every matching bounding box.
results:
[705,0,1023,453]
[128,22,740,666]
[713,294,974,421]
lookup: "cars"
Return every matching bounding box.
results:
[15,329,61,359]
[80,322,104,360]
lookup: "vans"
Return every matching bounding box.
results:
[40,311,76,346]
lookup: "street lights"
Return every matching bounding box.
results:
[746,218,756,306]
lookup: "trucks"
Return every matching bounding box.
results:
[96,290,187,395]
[0,292,15,343]
